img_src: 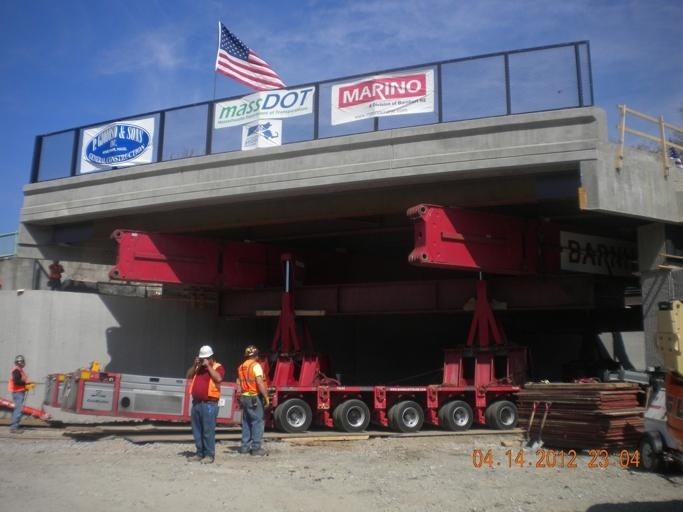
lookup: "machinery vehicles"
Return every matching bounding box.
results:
[37,201,639,431]
[632,293,683,469]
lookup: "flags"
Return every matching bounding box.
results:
[215,22,286,90]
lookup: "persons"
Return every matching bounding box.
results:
[48,260,63,289]
[8,355,30,434]
[188,345,225,464]
[236,345,269,456]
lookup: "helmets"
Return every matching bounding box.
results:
[197,344,215,359]
[243,344,259,358]
[14,354,24,363]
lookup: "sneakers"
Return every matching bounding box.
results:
[240,445,265,456]
[187,454,201,462]
[201,455,215,465]
[9,427,25,434]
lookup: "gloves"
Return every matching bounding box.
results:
[262,395,270,408]
